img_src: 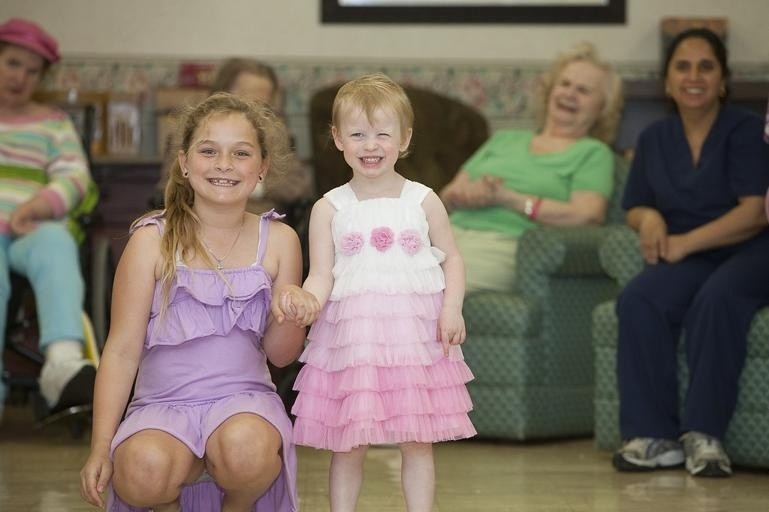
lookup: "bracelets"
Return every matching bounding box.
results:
[529,198,544,219]
[525,196,538,216]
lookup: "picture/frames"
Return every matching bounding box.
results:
[319,1,627,27]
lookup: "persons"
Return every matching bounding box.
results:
[80,92,321,512]
[611,28,769,477]
[154,59,310,215]
[365,43,625,449]
[279,72,478,512]
[0,19,96,414]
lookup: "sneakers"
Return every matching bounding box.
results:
[37,357,97,414]
[612,436,684,470]
[678,430,732,477]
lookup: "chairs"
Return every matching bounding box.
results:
[592,222,769,469]
[463,130,632,443]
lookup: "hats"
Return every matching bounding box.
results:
[1,17,60,63]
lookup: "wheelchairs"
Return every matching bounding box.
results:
[0,265,111,443]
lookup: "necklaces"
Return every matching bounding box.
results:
[188,212,245,269]
